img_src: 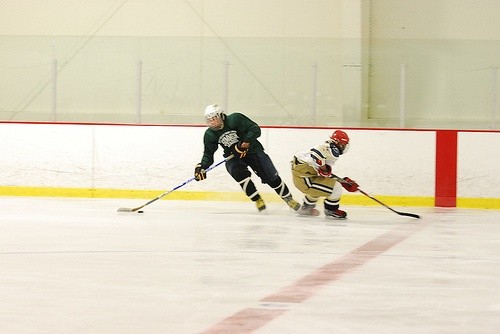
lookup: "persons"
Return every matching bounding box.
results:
[291,130,359,217]
[194,105,302,213]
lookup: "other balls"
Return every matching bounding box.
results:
[138,211,143,213]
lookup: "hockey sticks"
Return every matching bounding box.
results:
[118,153,235,213]
[330,173,420,218]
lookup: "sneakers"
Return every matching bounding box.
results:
[286,197,301,214]
[297,202,320,218]
[255,195,267,214]
[324,206,347,219]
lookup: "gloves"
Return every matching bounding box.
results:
[316,159,333,178]
[231,141,249,159]
[194,162,207,182]
[340,176,360,193]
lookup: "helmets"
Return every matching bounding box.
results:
[330,130,350,147]
[202,103,225,120]
